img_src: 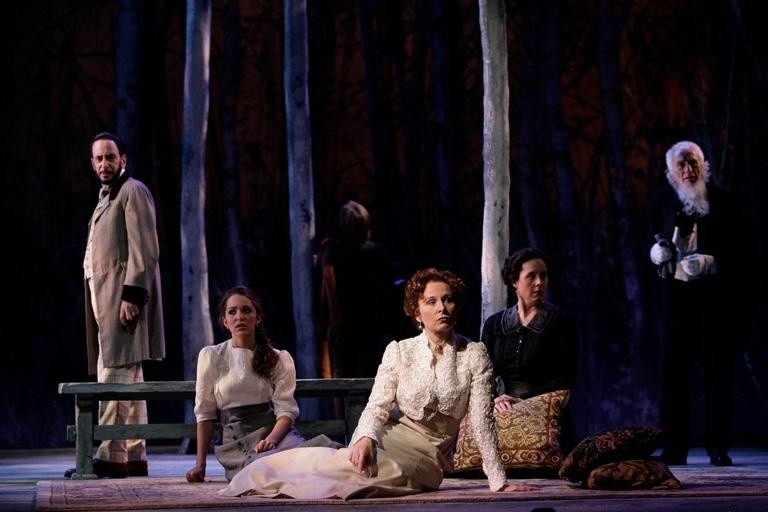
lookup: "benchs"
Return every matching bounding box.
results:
[55,375,377,483]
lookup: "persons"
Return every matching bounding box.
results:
[318,200,378,419]
[185,286,344,482]
[64,132,165,479]
[218,268,540,500]
[642,139,742,466]
[480,249,574,478]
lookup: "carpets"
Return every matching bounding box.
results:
[29,462,767,512]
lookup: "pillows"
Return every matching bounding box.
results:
[567,457,684,492]
[447,386,571,477]
[554,422,670,480]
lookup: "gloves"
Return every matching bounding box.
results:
[680,253,705,277]
[648,238,672,265]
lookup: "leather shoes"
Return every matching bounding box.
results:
[127,460,148,477]
[64,459,127,478]
[710,454,731,466]
